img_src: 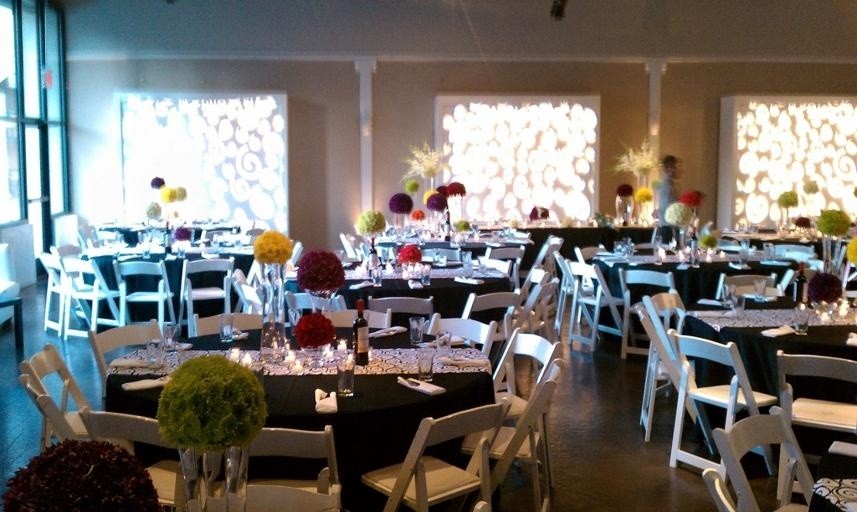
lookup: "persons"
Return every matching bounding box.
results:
[657,154,683,225]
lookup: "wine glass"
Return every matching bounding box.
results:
[302,347,325,368]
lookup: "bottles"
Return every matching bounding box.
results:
[351,299,370,365]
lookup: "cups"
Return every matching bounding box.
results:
[512,215,656,230]
[88,222,253,258]
[436,330,451,359]
[334,351,356,397]
[610,225,790,265]
[142,311,233,373]
[724,259,856,337]
[337,212,510,288]
[286,307,301,333]
[409,317,424,346]
[417,355,433,383]
